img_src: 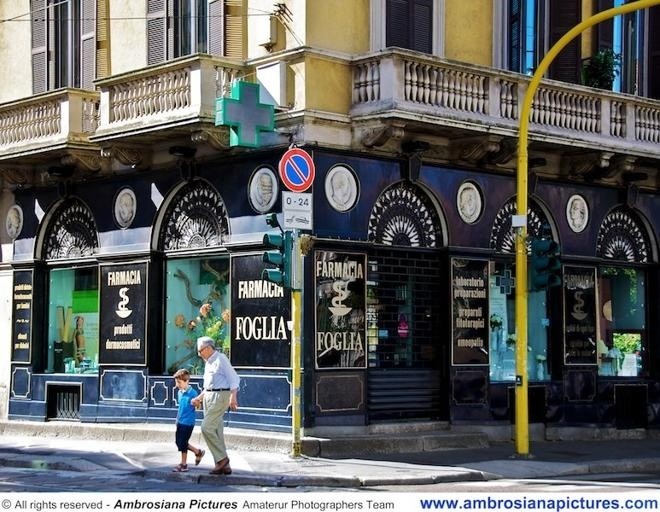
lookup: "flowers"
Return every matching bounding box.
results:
[613,333,640,353]
[507,334,515,352]
[490,314,502,331]
[536,355,546,360]
[176,304,229,350]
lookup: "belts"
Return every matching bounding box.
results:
[203,388,230,392]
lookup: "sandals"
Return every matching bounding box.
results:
[194,448,205,466]
[172,463,189,472]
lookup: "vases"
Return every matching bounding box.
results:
[537,360,544,380]
[617,354,638,377]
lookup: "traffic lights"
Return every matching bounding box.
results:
[260,226,293,291]
[527,237,555,269]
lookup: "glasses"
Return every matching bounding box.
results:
[198,346,207,353]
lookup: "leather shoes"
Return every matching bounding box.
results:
[209,467,232,475]
[212,457,229,472]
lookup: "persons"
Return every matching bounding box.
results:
[5,208,21,238]
[172,368,206,474]
[189,336,241,475]
[568,199,587,229]
[255,173,274,208]
[119,193,134,223]
[71,316,87,365]
[460,187,480,218]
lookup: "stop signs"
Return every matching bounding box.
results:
[396,321,410,339]
